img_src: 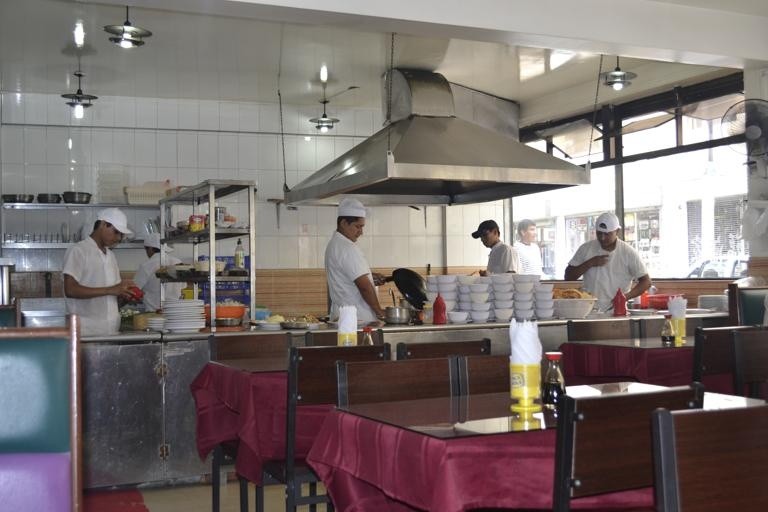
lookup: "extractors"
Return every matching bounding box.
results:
[281,64,591,206]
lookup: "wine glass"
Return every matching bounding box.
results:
[508,362,545,414]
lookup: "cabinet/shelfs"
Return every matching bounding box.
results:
[159,179,257,331]
[1,202,163,248]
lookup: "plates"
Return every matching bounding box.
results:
[696,294,727,311]
[249,316,364,330]
[146,299,205,332]
[627,308,710,315]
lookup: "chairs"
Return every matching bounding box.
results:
[0,315,81,512]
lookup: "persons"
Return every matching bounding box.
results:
[513,219,545,279]
[472,220,517,276]
[64,207,138,336]
[565,213,651,315]
[324,197,385,324]
[134,233,187,312]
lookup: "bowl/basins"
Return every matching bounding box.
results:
[36,193,61,202]
[198,262,227,272]
[553,298,598,319]
[384,306,409,324]
[2,194,34,203]
[205,305,247,326]
[644,293,684,308]
[61,191,90,203]
[248,310,271,320]
[423,272,555,325]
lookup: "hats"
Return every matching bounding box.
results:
[94,206,132,235]
[144,233,175,254]
[337,197,367,219]
[471,219,500,239]
[596,212,622,234]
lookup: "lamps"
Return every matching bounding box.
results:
[61,72,99,121]
[598,56,637,91]
[103,5,152,48]
[308,101,340,132]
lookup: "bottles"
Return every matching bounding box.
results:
[361,328,374,345]
[543,352,566,406]
[660,315,675,344]
[234,238,245,268]
[613,287,625,315]
[627,283,656,309]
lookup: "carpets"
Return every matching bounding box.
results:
[81,486,148,512]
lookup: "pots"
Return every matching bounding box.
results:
[382,268,427,310]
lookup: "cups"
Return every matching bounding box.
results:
[670,319,687,344]
[188,206,236,232]
[3,232,64,243]
[510,412,540,432]
[337,332,357,347]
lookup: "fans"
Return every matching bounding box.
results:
[720,99,768,179]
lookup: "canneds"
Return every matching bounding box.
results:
[215,207,225,226]
[189,215,205,232]
[181,289,193,300]
